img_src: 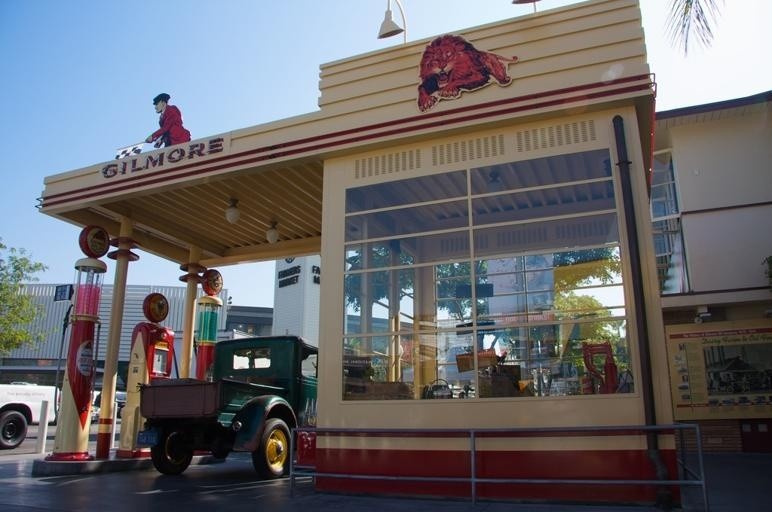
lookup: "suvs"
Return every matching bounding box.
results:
[94,391,127,419]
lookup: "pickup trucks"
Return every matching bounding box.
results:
[1,384,61,448]
[140,335,318,479]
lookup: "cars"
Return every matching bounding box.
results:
[11,381,37,385]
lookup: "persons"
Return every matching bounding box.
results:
[144,93,191,147]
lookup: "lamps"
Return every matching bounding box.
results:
[225,198,240,224]
[377,0,406,43]
[512,0,541,13]
[265,221,279,244]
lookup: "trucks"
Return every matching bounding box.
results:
[170,328,271,383]
